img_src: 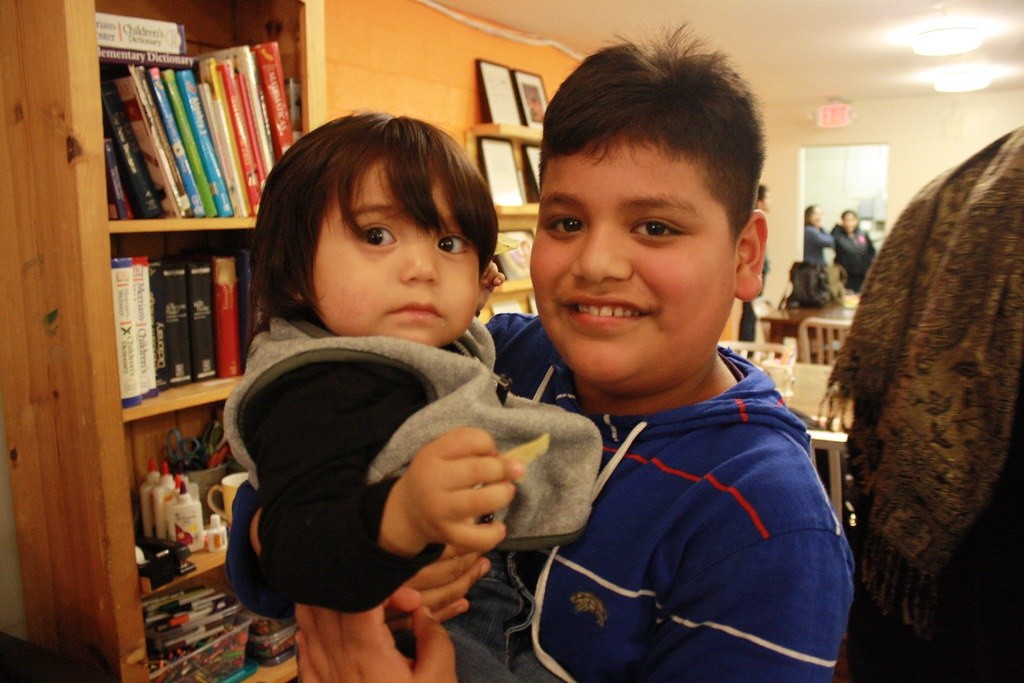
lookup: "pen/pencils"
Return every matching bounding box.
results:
[146,548,169,561]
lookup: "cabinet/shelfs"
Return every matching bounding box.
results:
[0,0,329,682]
[473,122,542,293]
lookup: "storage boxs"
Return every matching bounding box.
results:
[150,612,253,683]
[247,614,296,658]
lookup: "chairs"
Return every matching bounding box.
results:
[718,335,796,364]
[798,316,854,364]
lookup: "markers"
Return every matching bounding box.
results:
[142,585,249,683]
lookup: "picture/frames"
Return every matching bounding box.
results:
[490,301,522,317]
[512,69,549,128]
[476,135,523,205]
[522,144,542,195]
[475,59,524,125]
[527,294,538,314]
[493,229,534,280]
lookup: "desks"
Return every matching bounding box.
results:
[760,305,858,360]
[755,361,854,431]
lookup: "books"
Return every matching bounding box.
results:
[110,248,251,409]
[96,11,295,219]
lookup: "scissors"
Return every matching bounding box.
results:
[162,418,228,472]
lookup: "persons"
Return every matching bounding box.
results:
[222,38,879,683]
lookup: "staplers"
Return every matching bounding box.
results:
[136,534,197,576]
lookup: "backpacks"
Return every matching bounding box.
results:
[778,262,832,311]
